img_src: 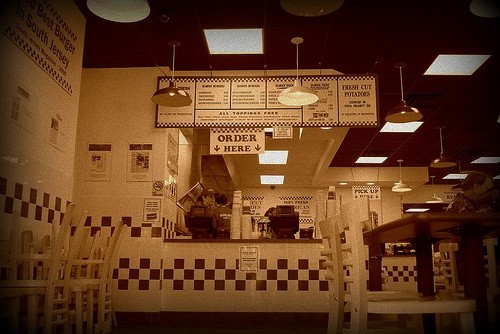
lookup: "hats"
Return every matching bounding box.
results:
[201,188,217,196]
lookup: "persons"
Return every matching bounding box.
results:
[211,192,232,239]
[202,188,215,209]
[446,171,500,213]
[264,207,277,217]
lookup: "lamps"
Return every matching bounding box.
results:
[428,127,457,168]
[151,41,193,106]
[468,0,500,18]
[280,0,342,17]
[392,158,412,193]
[279,37,319,105]
[425,175,445,204]
[385,63,422,123]
[86,0,151,23]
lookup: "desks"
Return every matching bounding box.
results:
[363,212,500,334]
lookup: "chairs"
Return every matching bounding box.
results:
[1,200,131,334]
[318,196,500,333]
